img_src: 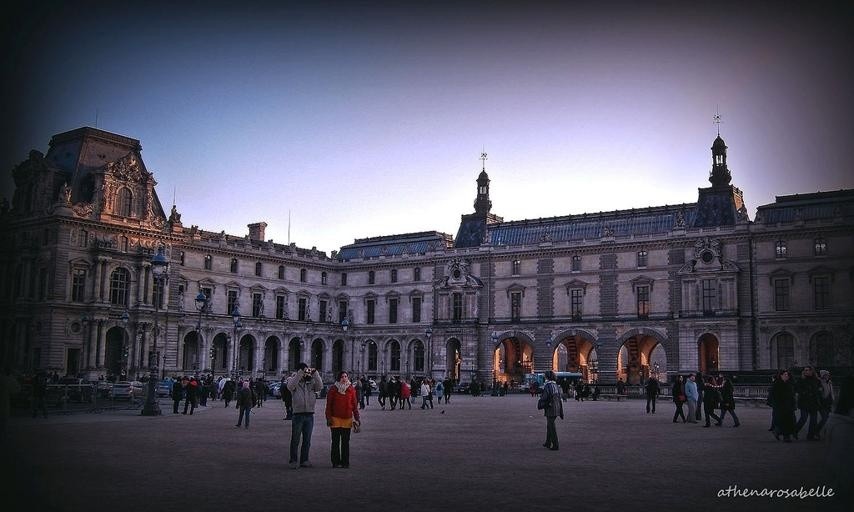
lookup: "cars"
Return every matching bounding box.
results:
[505,381,525,392]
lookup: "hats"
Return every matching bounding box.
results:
[819,370,830,378]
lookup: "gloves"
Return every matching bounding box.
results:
[326,419,335,427]
[355,417,362,426]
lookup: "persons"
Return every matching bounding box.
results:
[1,362,483,470]
[491,365,835,449]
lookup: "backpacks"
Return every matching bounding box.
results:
[436,382,443,391]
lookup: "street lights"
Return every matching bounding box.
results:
[230,303,242,379]
[424,325,432,378]
[340,310,350,376]
[655,361,661,380]
[589,360,599,383]
[194,287,208,374]
[639,364,646,397]
[140,244,170,414]
[76,315,89,378]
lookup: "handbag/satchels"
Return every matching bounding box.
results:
[537,397,549,410]
[426,394,433,400]
[678,393,687,402]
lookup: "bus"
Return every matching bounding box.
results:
[525,371,585,392]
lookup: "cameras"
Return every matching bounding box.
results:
[306,368,312,373]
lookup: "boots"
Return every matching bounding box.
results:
[174,402,207,415]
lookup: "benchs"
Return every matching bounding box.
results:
[588,394,629,402]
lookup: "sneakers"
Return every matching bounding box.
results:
[358,400,451,411]
[235,424,250,430]
[220,397,229,408]
[768,428,822,442]
[287,460,349,469]
[283,415,292,420]
[646,409,741,428]
[542,442,559,451]
